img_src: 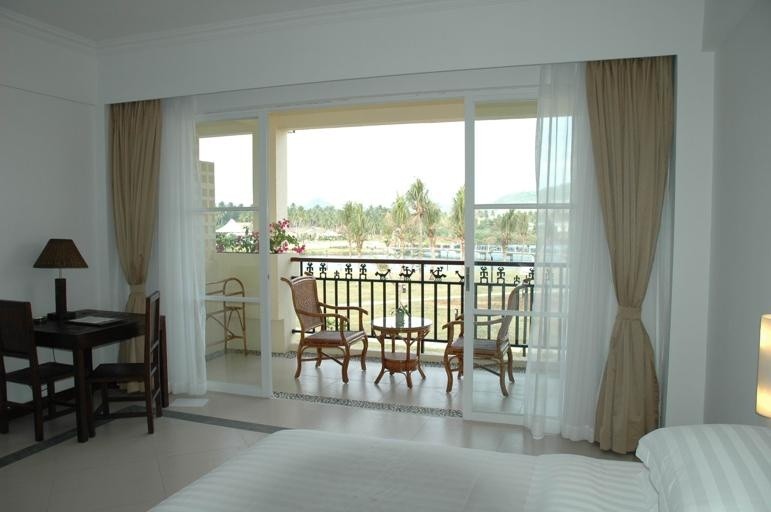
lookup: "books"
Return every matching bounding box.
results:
[68,314,127,327]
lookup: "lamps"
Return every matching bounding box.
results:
[755,310,770,423]
[32,238,91,319]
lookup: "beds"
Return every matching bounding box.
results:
[144,420,770,510]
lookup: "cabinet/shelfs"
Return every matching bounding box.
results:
[204,276,249,361]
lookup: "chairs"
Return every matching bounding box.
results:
[441,278,533,397]
[280,271,370,384]
[0,298,88,441]
[90,290,164,438]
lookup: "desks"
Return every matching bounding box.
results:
[21,305,170,444]
[369,315,434,390]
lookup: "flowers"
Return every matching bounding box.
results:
[216,217,308,255]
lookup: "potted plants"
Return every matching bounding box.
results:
[387,303,411,325]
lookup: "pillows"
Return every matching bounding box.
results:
[633,422,770,510]
